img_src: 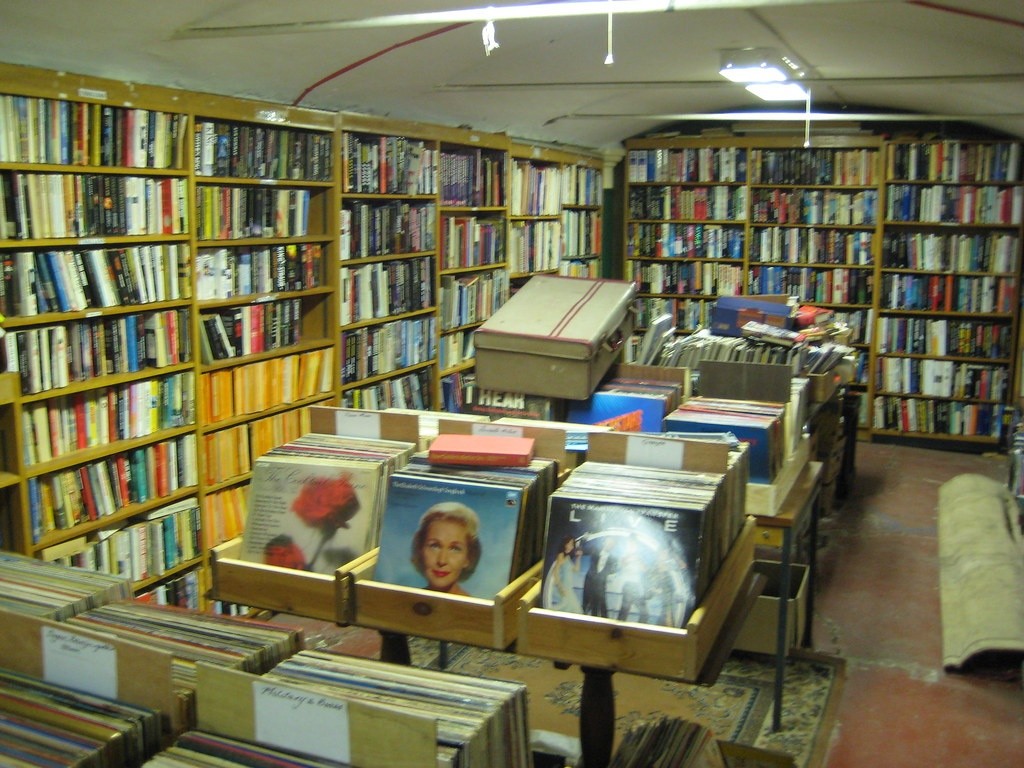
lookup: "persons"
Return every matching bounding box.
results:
[552,535,584,613]
[411,501,480,598]
[617,533,654,625]
[653,550,686,628]
[580,532,621,616]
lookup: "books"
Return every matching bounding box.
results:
[0,92,601,617]
[624,140,1019,188]
[544,224,874,627]
[0,551,726,768]
[628,186,877,224]
[874,184,1022,438]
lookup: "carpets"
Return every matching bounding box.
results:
[369,635,847,768]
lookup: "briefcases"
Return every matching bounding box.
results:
[473,274,641,401]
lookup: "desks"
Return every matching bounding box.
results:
[745,459,828,732]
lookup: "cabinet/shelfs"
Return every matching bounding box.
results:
[869,139,1024,445]
[340,110,513,413]
[510,142,605,299]
[0,61,340,623]
[624,136,884,441]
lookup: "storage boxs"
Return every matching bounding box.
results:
[731,558,810,657]
